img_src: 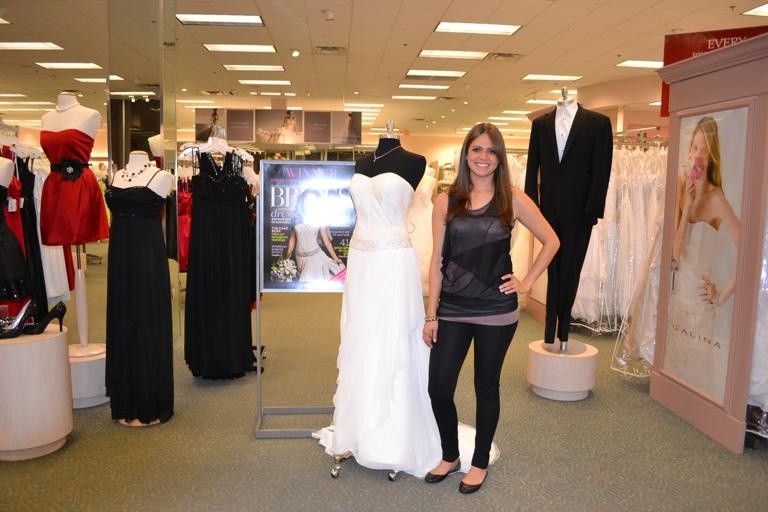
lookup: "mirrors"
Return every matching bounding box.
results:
[107,0,163,174]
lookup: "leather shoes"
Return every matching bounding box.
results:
[458,468,488,496]
[425,458,461,484]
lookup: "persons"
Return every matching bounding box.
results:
[195,109,224,141]
[39,91,110,246]
[102,150,176,427]
[311,137,500,479]
[333,113,359,144]
[523,98,613,351]
[664,115,742,406]
[423,122,561,494]
[148,133,161,168]
[258,110,301,145]
[0,157,30,303]
[286,189,343,281]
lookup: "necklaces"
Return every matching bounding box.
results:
[372,146,401,162]
[120,164,151,183]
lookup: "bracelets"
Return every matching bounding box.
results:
[425,318,438,323]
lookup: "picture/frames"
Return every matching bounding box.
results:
[656,95,758,411]
